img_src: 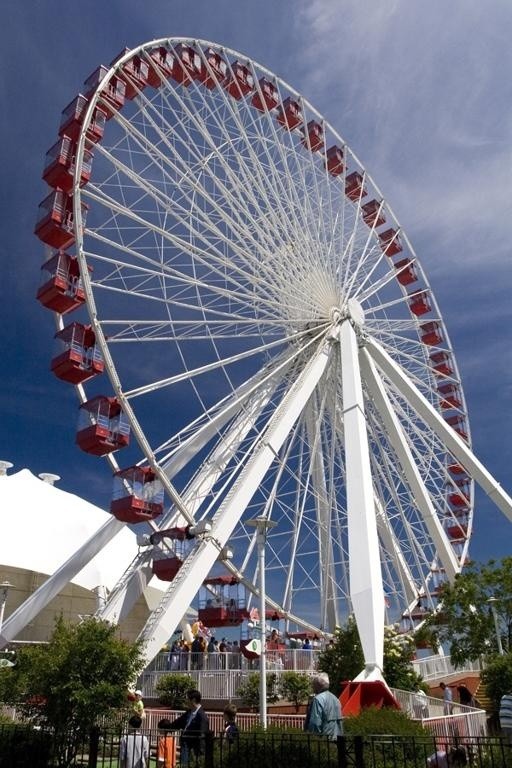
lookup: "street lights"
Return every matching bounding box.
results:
[484,597,505,654]
[246,516,278,730]
[0,580,15,626]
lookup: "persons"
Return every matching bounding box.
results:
[412,683,429,717]
[132,690,144,717]
[427,746,467,768]
[169,689,209,763]
[457,683,471,711]
[221,705,240,745]
[439,682,452,716]
[170,636,243,671]
[206,597,236,614]
[120,715,151,768]
[499,688,512,738]
[156,720,176,768]
[265,627,337,654]
[307,672,343,742]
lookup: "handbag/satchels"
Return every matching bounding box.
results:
[139,709,147,719]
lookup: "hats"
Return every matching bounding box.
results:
[134,690,143,698]
